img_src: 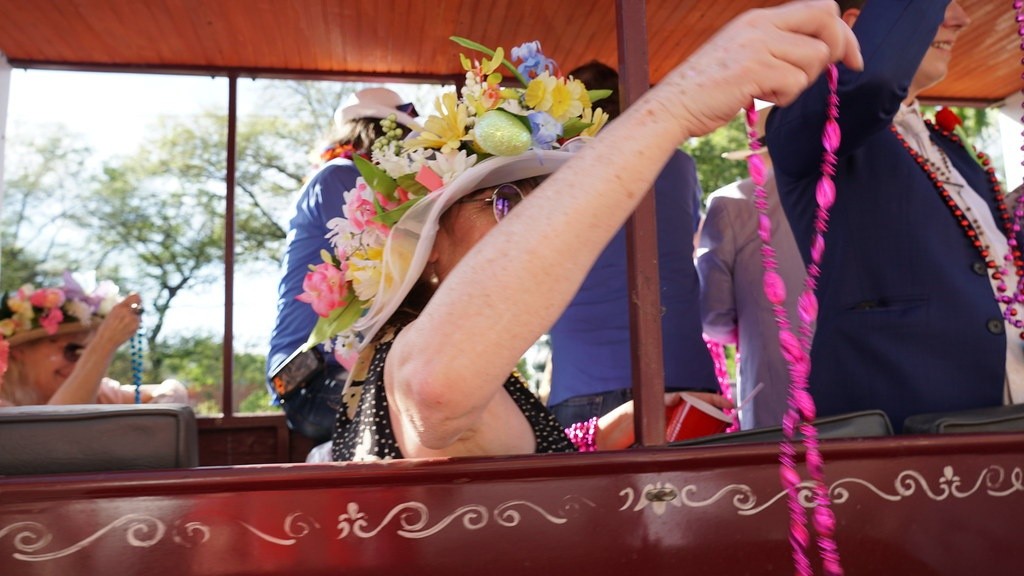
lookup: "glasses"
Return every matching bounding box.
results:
[50,341,84,361]
[461,182,523,224]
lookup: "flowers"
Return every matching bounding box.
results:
[0,266,133,376]
[936,106,988,170]
[292,31,613,370]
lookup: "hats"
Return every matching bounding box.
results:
[0,277,105,374]
[335,89,424,132]
[353,128,587,351]
[746,102,776,143]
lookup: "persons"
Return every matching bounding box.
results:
[695,103,819,431]
[270,87,418,440]
[544,58,720,426]
[0,288,187,405]
[268,0,863,462]
[766,0,1023,435]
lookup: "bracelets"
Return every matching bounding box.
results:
[565,416,599,453]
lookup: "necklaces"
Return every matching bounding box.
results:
[888,1,1023,339]
[130,304,145,405]
[321,142,368,164]
[704,321,740,434]
[747,63,848,575]
